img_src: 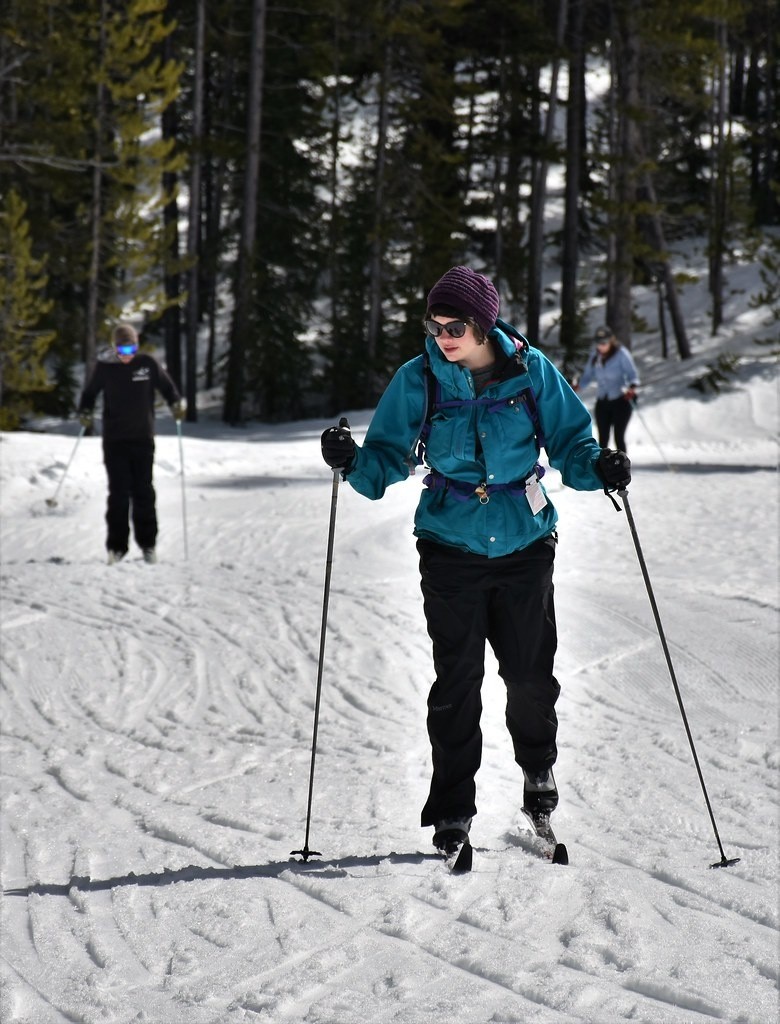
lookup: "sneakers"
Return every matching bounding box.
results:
[431,815,472,848]
[141,547,157,564]
[521,765,559,814]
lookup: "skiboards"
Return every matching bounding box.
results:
[106,548,158,565]
[436,806,569,875]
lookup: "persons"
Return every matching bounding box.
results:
[321,265,632,855]
[574,326,641,453]
[80,327,185,564]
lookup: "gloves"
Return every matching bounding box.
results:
[77,408,92,426]
[593,447,631,490]
[625,389,634,400]
[321,419,356,468]
[565,378,579,392]
[170,397,188,419]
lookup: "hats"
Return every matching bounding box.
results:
[425,265,500,331]
[110,325,139,343]
[594,326,612,343]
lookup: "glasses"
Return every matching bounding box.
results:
[116,344,138,355]
[424,319,469,338]
